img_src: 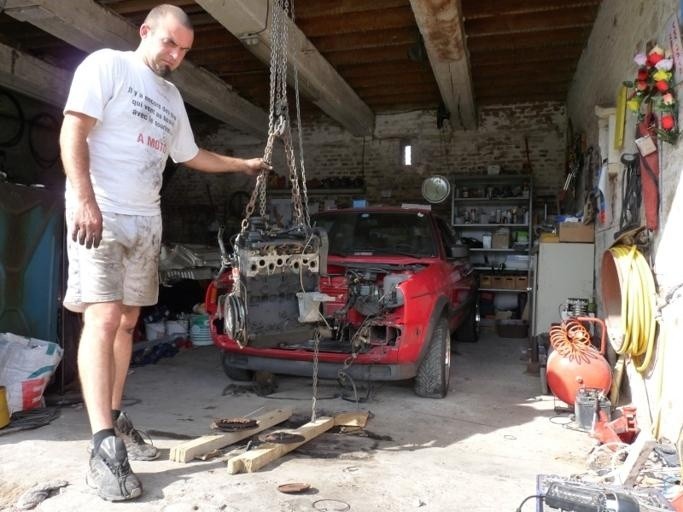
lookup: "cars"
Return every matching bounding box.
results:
[202,205,483,401]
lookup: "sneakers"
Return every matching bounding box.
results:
[85,436,142,502]
[113,412,159,461]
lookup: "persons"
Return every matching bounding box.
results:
[57,2,277,504]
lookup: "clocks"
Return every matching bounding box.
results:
[421,175,451,204]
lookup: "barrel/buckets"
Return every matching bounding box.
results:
[0,386,11,429]
[145,314,214,347]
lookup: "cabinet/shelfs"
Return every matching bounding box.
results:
[450,174,533,292]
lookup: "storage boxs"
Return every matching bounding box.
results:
[498,325,527,338]
[559,223,595,242]
[479,272,528,290]
